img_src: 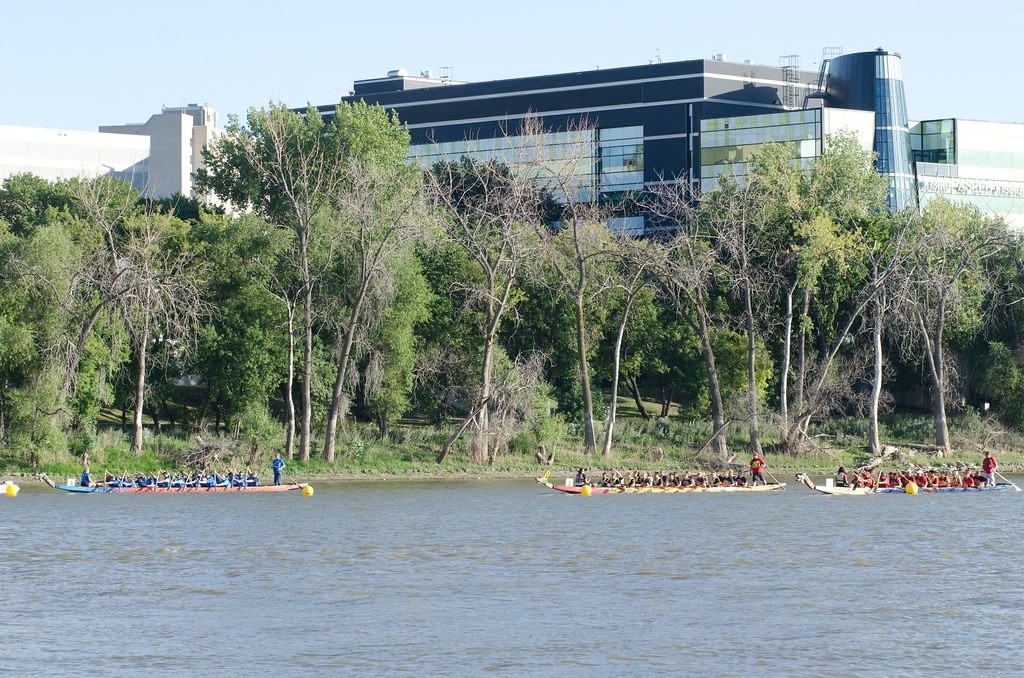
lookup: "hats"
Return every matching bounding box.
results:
[888,472,893,474]
[966,471,971,474]
[916,470,923,474]
[752,452,758,456]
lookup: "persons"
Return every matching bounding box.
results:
[576,469,748,488]
[750,451,767,487]
[81,467,260,487]
[836,466,989,488]
[982,452,998,486]
[270,453,285,485]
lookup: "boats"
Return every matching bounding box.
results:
[795,473,1015,494]
[536,471,785,493]
[42,473,310,494]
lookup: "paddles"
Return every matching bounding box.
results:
[849,446,1024,498]
[595,462,788,496]
[98,456,310,501]
